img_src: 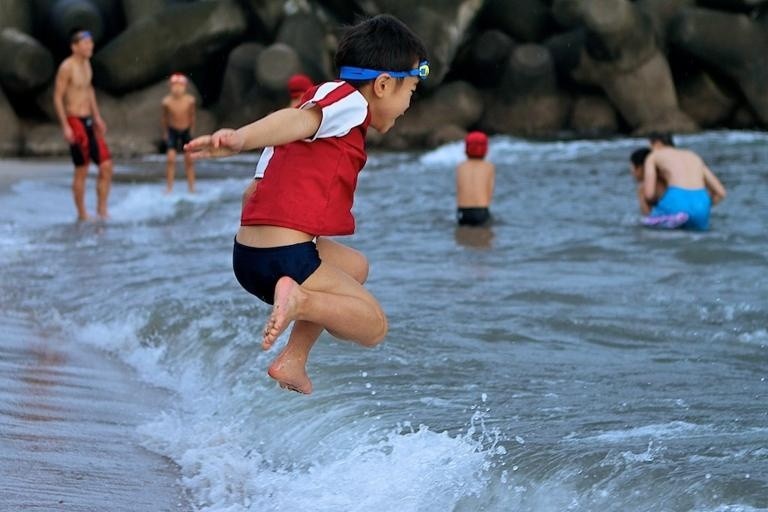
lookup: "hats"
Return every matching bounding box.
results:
[465,130,489,159]
[286,73,314,99]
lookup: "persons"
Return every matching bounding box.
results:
[161,73,196,193]
[455,130,495,226]
[288,75,313,106]
[630,148,664,214]
[183,13,432,394]
[643,130,726,232]
[52,29,112,218]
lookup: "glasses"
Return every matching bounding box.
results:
[339,60,429,81]
